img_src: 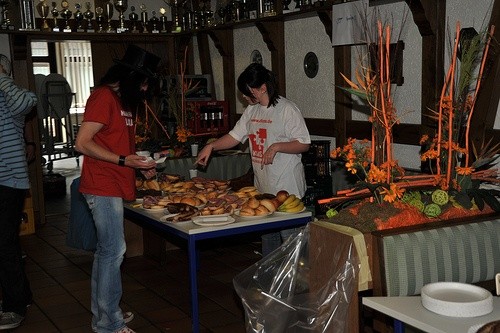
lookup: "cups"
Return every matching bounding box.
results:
[192,145,198,156]
[189,169,197,178]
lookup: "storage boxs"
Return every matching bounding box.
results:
[180,100,229,133]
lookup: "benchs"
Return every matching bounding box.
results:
[373,212,500,333]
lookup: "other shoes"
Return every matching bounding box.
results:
[121,312,134,323]
[0,300,26,330]
[110,325,136,333]
[251,288,270,301]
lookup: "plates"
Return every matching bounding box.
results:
[160,214,193,224]
[304,52,319,78]
[250,49,263,66]
[140,206,168,212]
[421,282,494,318]
[193,215,235,226]
[233,211,272,219]
[275,206,305,214]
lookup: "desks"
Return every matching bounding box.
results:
[362,297,500,333]
[123,199,312,333]
[158,152,251,181]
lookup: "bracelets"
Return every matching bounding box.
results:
[118,155,126,166]
[27,141,36,146]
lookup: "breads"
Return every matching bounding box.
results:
[134,175,275,216]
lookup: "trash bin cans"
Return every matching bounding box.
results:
[70,176,97,250]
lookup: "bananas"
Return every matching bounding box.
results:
[278,194,305,212]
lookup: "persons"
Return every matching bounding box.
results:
[193,63,311,301]
[75,69,160,333]
[0,54,38,330]
